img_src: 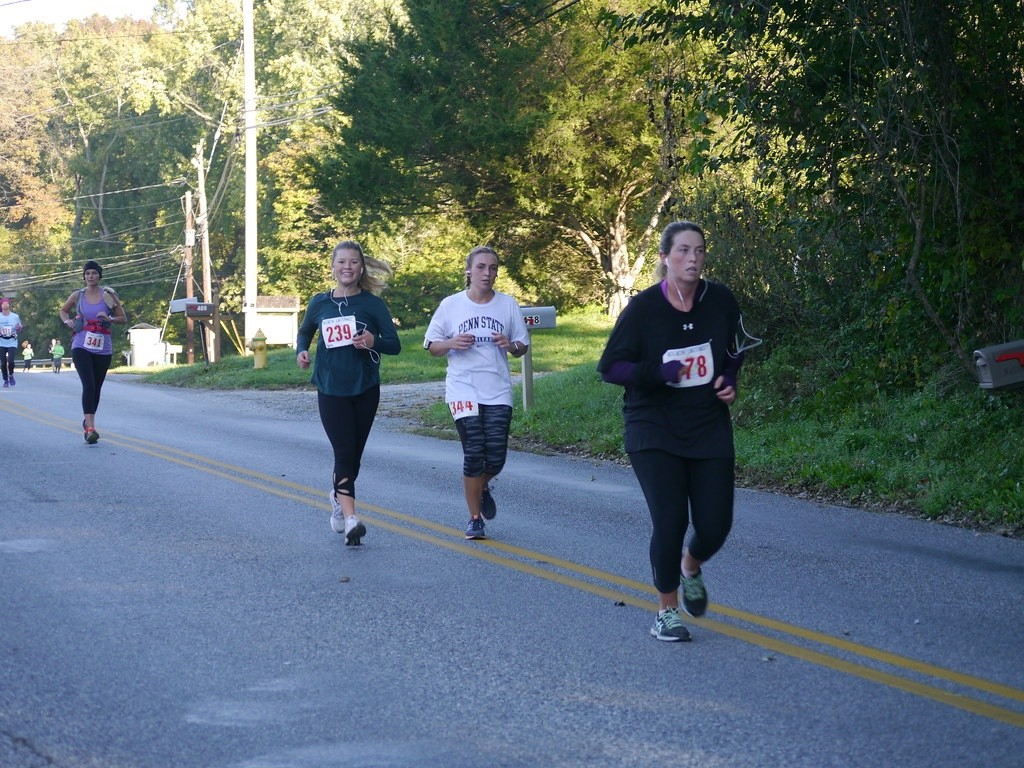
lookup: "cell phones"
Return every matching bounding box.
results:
[354,329,363,338]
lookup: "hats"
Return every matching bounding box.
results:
[83,260,102,277]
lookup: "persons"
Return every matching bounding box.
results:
[296,240,401,546]
[60,261,127,444]
[48,338,65,374]
[0,297,23,387]
[22,339,35,373]
[424,248,530,540]
[596,221,747,641]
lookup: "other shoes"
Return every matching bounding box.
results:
[4,381,9,387]
[9,376,15,385]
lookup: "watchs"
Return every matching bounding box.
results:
[109,314,114,322]
[512,341,520,353]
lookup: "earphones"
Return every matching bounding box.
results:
[467,271,470,274]
[360,267,364,275]
[665,258,670,267]
[332,267,335,274]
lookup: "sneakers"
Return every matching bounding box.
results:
[650,605,692,641]
[86,430,99,443]
[329,489,344,532]
[680,566,707,616]
[344,515,366,545]
[480,486,496,519]
[465,514,485,540]
[82,419,87,442]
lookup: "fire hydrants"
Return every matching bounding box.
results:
[245,328,267,368]
